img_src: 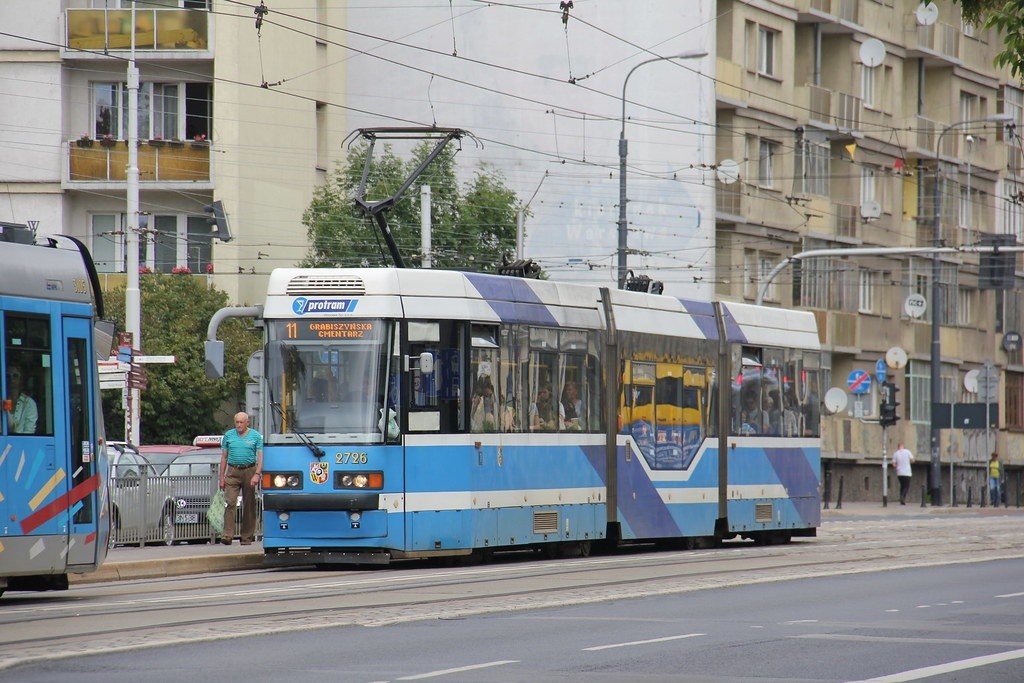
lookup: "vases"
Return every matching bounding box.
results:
[125,140,142,146]
[149,139,166,146]
[100,140,116,146]
[191,140,210,147]
[170,141,184,148]
[77,139,93,146]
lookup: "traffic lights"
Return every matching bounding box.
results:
[204,200,232,242]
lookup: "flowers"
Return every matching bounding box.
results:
[171,135,180,141]
[154,134,162,140]
[102,134,113,141]
[80,131,90,141]
[193,134,206,142]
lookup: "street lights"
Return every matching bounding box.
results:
[926,113,1015,507]
[615,48,710,291]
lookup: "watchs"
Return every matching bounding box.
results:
[255,472,262,478]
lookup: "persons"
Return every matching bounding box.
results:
[456,380,582,431]
[892,442,915,505]
[6,361,38,434]
[741,391,799,436]
[989,453,1004,507]
[339,369,395,411]
[219,411,263,545]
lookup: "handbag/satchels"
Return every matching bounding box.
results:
[205,488,225,537]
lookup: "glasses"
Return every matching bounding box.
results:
[6,372,21,379]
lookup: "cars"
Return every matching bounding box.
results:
[105,434,264,549]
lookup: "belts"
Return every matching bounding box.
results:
[228,463,255,469]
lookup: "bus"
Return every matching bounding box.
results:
[0,216,116,603]
[199,123,825,572]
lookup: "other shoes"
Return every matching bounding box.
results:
[240,541,251,545]
[221,536,232,545]
[900,495,906,505]
